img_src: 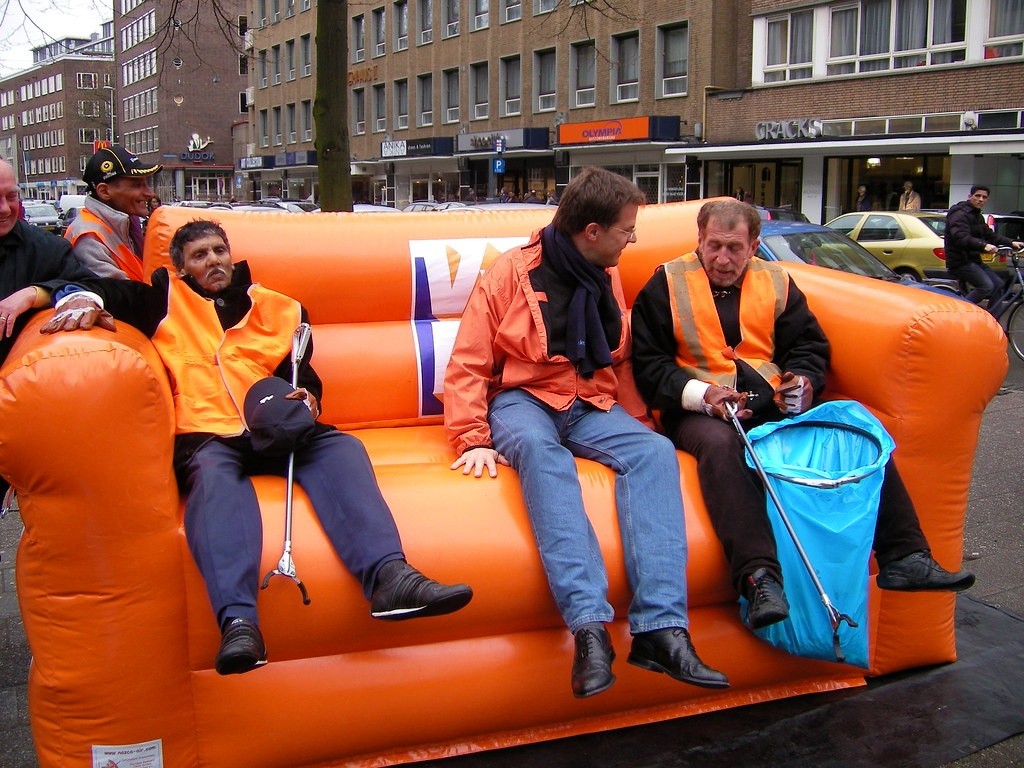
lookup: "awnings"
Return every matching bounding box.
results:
[36,183,45,187]
[20,184,27,188]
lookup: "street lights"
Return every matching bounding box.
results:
[103,84,115,147]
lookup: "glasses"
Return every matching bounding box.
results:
[598,222,637,239]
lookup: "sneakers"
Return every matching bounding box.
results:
[215,618,267,675]
[746,573,789,628]
[371,564,471,619]
[876,549,976,590]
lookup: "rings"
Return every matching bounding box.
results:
[1,316,6,320]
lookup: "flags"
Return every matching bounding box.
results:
[24,152,30,175]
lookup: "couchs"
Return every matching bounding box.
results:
[0,192,1009,768]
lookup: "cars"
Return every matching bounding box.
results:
[21,194,86,237]
[753,217,974,305]
[795,208,1008,284]
[751,204,810,223]
[845,210,1024,285]
[139,195,559,234]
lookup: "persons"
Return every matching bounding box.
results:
[447,190,460,202]
[945,183,1024,321]
[544,188,560,206]
[142,193,162,236]
[733,185,753,205]
[885,185,898,210]
[463,187,476,201]
[39,217,474,678]
[523,189,542,204]
[0,156,80,369]
[499,186,520,203]
[854,186,872,211]
[898,181,921,211]
[873,189,883,209]
[630,207,975,629]
[65,146,163,288]
[442,169,730,698]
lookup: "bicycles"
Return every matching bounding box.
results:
[921,243,1024,360]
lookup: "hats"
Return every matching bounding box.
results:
[243,377,315,452]
[81,146,164,191]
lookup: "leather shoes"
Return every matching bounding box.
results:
[571,623,616,698]
[627,625,729,688]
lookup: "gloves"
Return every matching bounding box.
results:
[702,385,754,422]
[776,371,814,415]
[40,291,117,335]
